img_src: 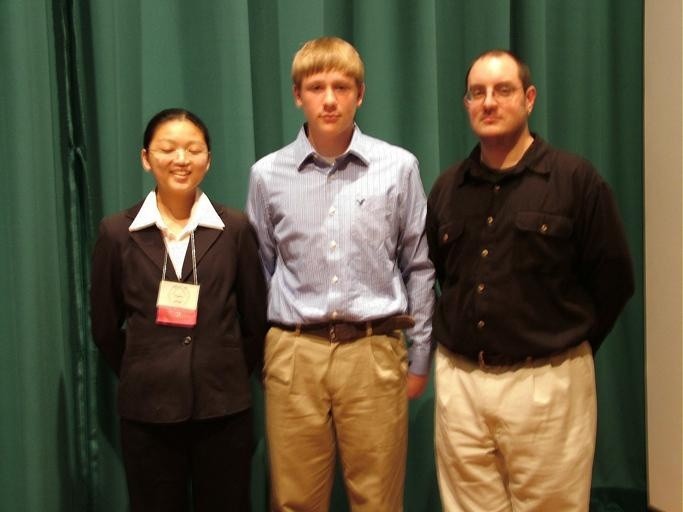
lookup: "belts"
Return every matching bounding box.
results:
[458,347,526,373]
[269,314,416,346]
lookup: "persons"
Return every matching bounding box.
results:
[424,47,633,512]
[245,35,436,510]
[88,107,266,512]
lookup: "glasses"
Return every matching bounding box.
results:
[463,85,529,105]
